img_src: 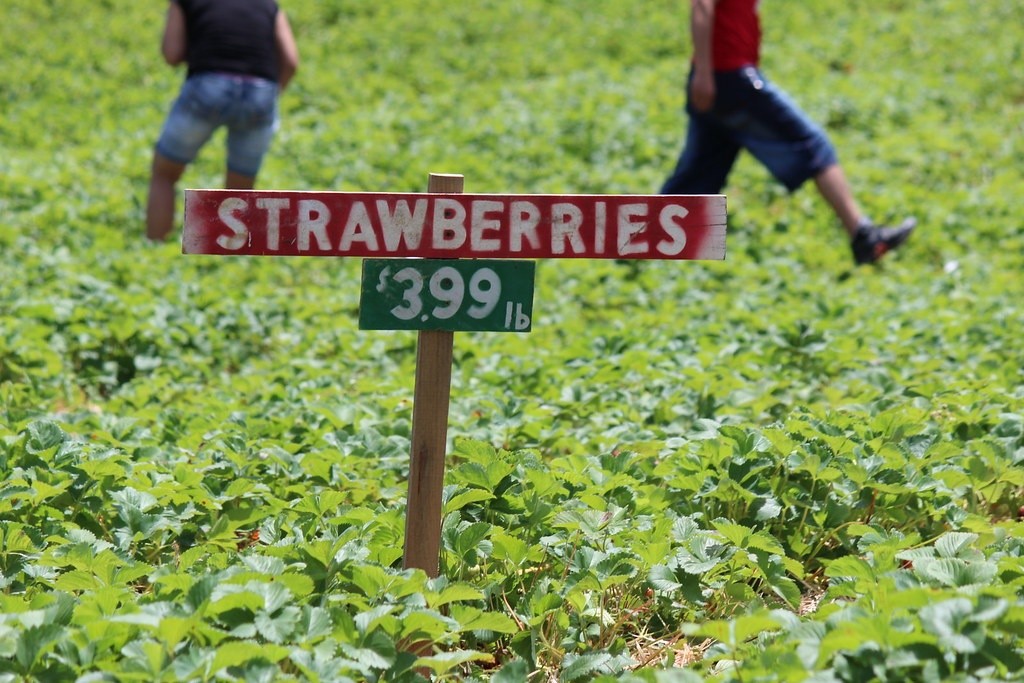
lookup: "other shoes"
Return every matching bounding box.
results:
[852,214,916,265]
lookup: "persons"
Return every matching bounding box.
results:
[659,0,918,266]
[146,0,298,241]
